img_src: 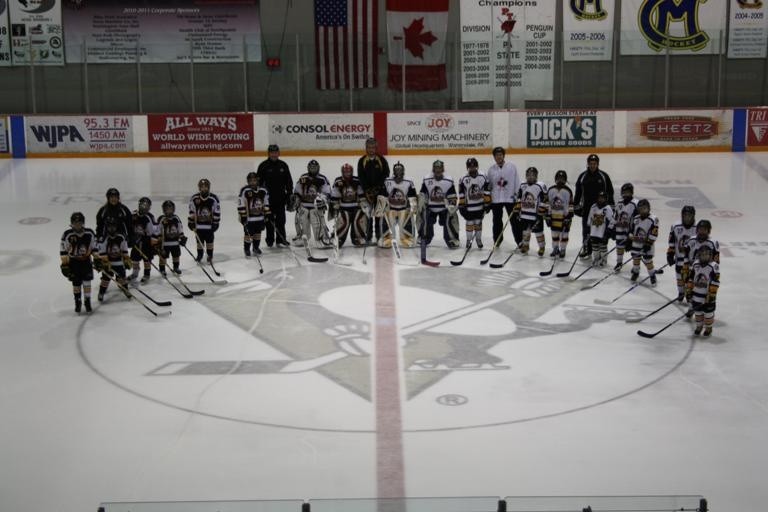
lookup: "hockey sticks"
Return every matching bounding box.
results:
[638,303,704,338]
[625,292,687,322]
[489,219,541,268]
[383,210,401,260]
[107,267,172,307]
[580,249,644,291]
[317,202,353,266]
[420,205,441,267]
[296,204,329,263]
[557,233,589,276]
[539,218,567,276]
[152,245,205,295]
[104,210,696,339]
[184,245,228,286]
[244,223,264,274]
[362,210,373,264]
[479,209,513,265]
[92,265,172,320]
[563,239,627,282]
[131,243,194,299]
[594,258,676,305]
[268,216,302,268]
[450,210,487,265]
[193,231,228,277]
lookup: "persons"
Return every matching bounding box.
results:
[61,188,187,317]
[186,177,222,267]
[237,141,573,259]
[573,154,657,287]
[666,205,721,340]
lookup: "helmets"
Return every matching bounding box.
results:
[525,167,568,183]
[307,159,354,176]
[71,212,84,233]
[466,159,478,178]
[393,162,405,178]
[106,188,175,233]
[247,171,259,184]
[588,155,600,165]
[433,160,444,167]
[198,179,210,195]
[493,147,505,157]
[683,204,713,265]
[366,138,377,151]
[595,183,650,221]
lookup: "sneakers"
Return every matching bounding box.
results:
[195,255,213,261]
[123,267,182,298]
[677,293,712,336]
[244,238,290,255]
[476,239,483,247]
[519,246,565,258]
[466,239,471,247]
[632,273,639,281]
[650,274,656,284]
[74,294,104,312]
[579,251,607,266]
[615,264,622,271]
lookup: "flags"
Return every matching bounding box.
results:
[386,0,450,92]
[314,0,378,92]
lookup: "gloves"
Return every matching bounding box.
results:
[61,264,71,277]
[121,254,134,269]
[94,256,111,273]
[459,203,491,214]
[240,217,246,224]
[265,213,270,222]
[643,245,650,252]
[625,239,632,252]
[189,222,196,230]
[514,205,582,226]
[211,223,219,232]
[667,253,676,266]
[180,233,188,247]
[682,269,715,305]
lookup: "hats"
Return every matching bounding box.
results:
[268,144,279,151]
